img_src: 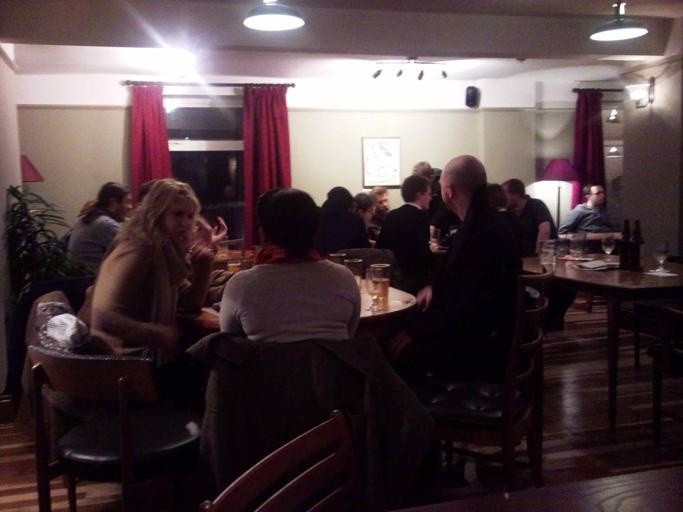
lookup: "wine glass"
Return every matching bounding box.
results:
[365,268,384,311]
[652,239,670,271]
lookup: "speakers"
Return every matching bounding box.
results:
[466,86,477,107]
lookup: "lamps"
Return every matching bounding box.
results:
[606,146,622,158]
[590,0,648,41]
[625,83,655,108]
[243,0,306,32]
[541,160,582,231]
[606,108,620,124]
[21,154,43,182]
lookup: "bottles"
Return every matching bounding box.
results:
[602,233,615,261]
[618,219,631,271]
[630,216,645,271]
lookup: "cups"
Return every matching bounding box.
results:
[226,260,242,274]
[343,259,364,288]
[538,229,586,266]
[330,254,347,264]
[370,263,390,307]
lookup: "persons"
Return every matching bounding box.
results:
[66,177,229,368]
[391,154,525,397]
[557,182,626,256]
[490,179,558,257]
[217,185,362,343]
[51,178,229,299]
[319,163,462,295]
